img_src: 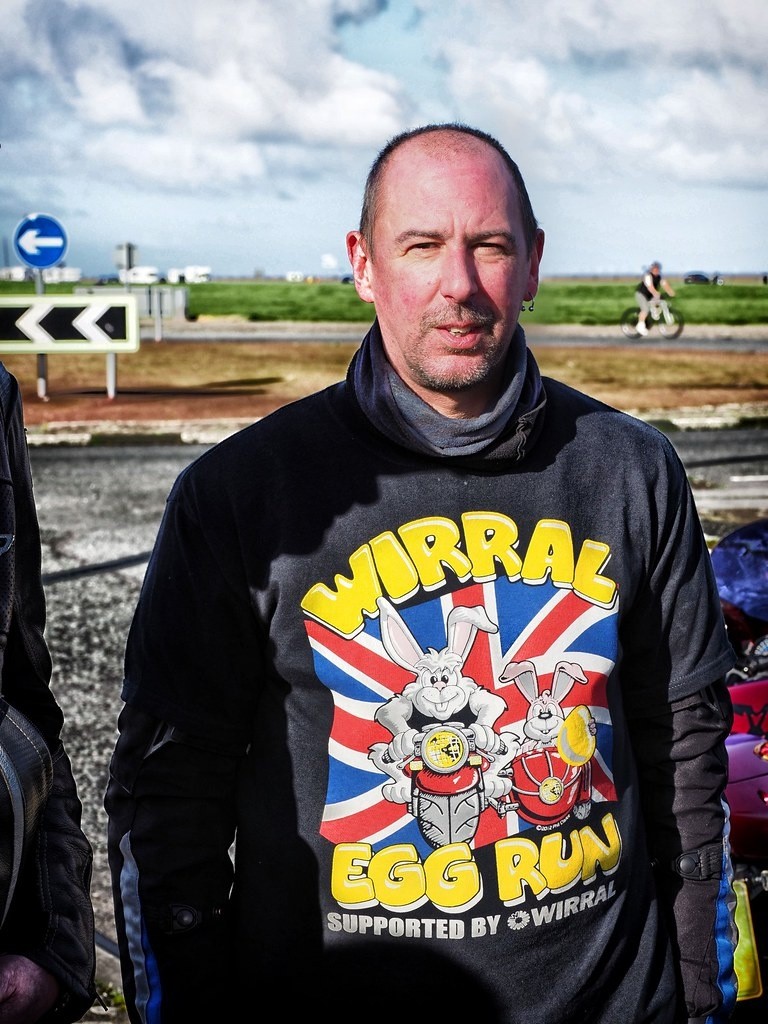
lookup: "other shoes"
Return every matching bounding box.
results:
[636,322,648,336]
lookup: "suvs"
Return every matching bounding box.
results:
[684,273,725,287]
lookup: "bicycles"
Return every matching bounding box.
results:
[620,292,684,339]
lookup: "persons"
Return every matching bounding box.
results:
[0,360,96,1024]
[103,121,737,1024]
[633,261,675,336]
